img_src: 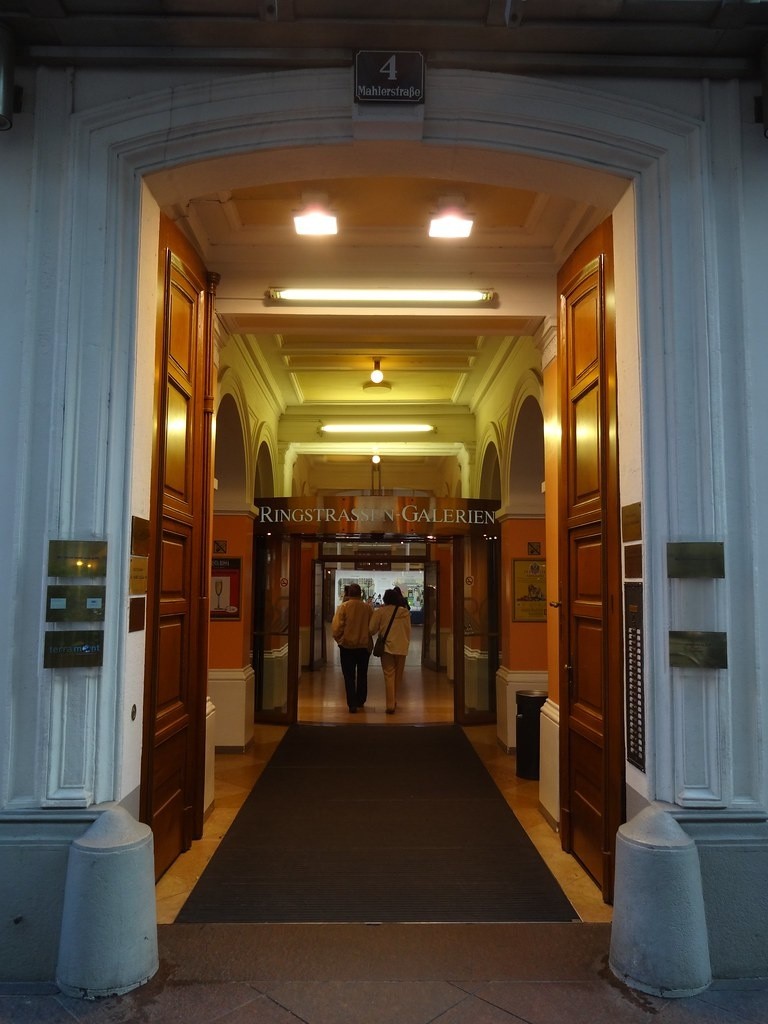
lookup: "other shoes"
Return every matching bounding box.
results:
[385,703,396,715]
[349,703,364,713]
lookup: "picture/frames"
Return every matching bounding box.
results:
[210,555,243,622]
[511,557,547,623]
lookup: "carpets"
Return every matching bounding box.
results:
[172,722,585,923]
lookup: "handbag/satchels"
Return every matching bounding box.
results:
[373,635,385,657]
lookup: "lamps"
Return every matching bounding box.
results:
[425,193,476,238]
[316,424,438,437]
[291,188,340,235]
[372,456,381,464]
[263,286,497,305]
[371,357,384,383]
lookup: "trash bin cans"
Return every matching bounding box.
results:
[514,689,548,780]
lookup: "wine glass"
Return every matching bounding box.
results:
[214,581,224,610]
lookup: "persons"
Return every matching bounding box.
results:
[332,583,412,714]
